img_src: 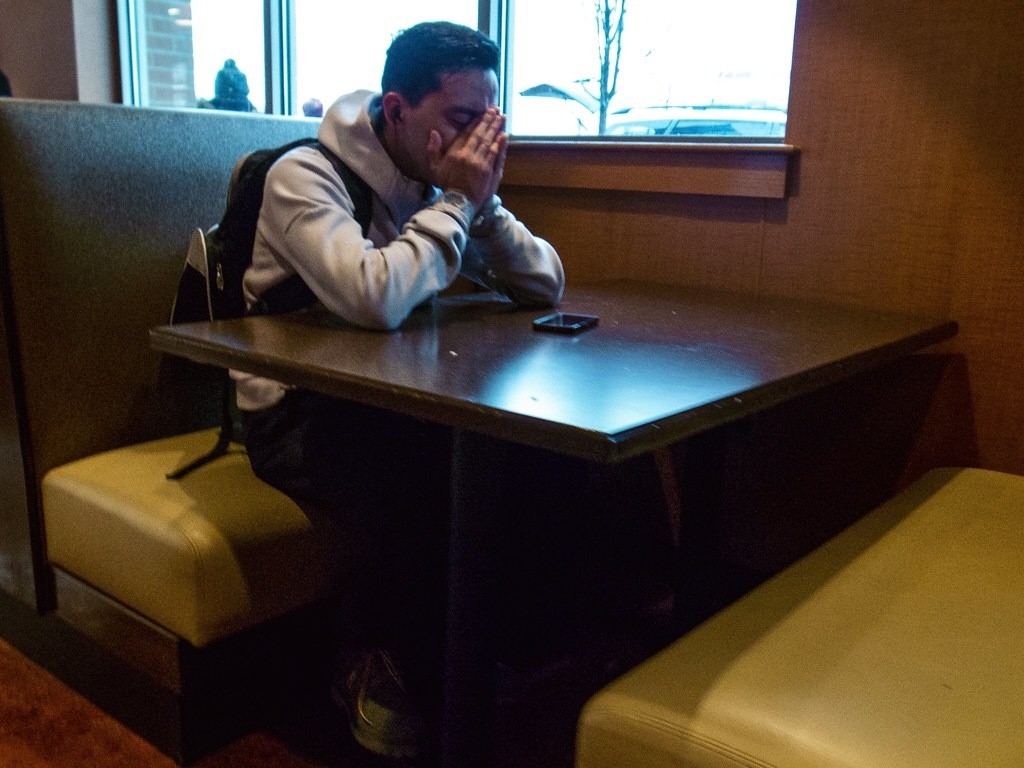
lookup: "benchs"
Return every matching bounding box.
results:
[577,469,1024,768]
[0,97,355,767]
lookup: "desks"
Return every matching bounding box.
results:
[150,278,959,768]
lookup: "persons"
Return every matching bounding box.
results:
[227,22,681,756]
[198,59,259,113]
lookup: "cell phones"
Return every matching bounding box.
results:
[531,312,599,334]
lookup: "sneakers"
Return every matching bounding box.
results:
[334,631,430,759]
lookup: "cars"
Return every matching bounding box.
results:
[603,103,790,137]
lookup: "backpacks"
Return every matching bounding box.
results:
[168,136,372,374]
